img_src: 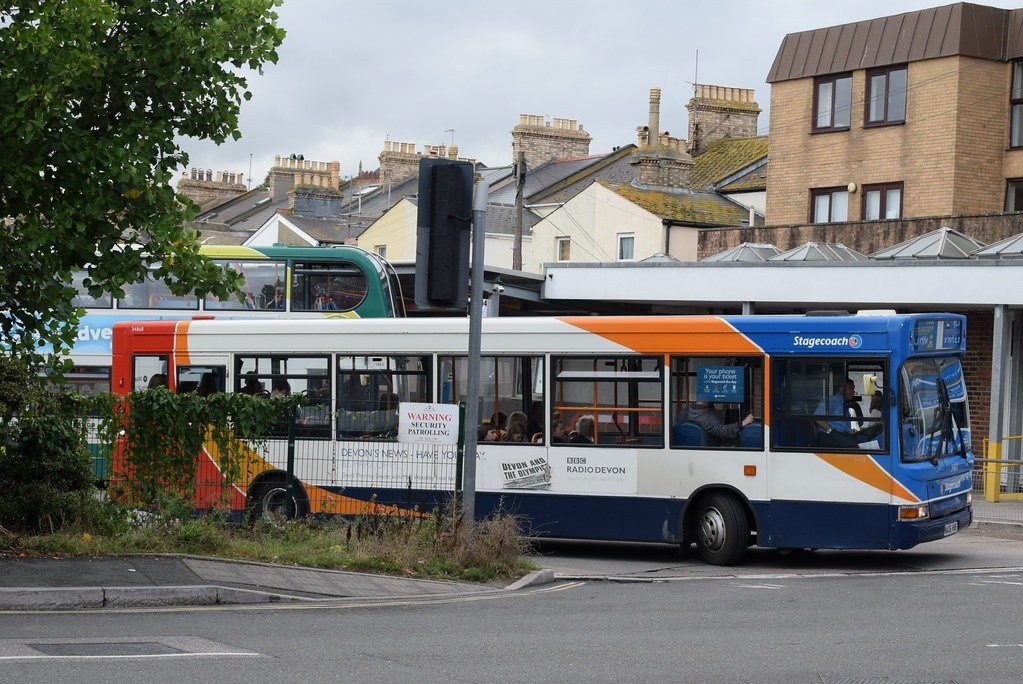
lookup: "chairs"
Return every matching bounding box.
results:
[532,432,543,444]
[803,398,821,447]
[672,422,707,446]
[567,430,578,443]
[739,423,761,448]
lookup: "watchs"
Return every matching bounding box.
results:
[827,429,832,434]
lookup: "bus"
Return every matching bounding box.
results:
[0,243,407,480]
[105,308,976,574]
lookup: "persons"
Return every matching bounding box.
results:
[868,390,883,427]
[688,401,754,447]
[241,369,399,430]
[477,396,595,444]
[812,377,861,447]
